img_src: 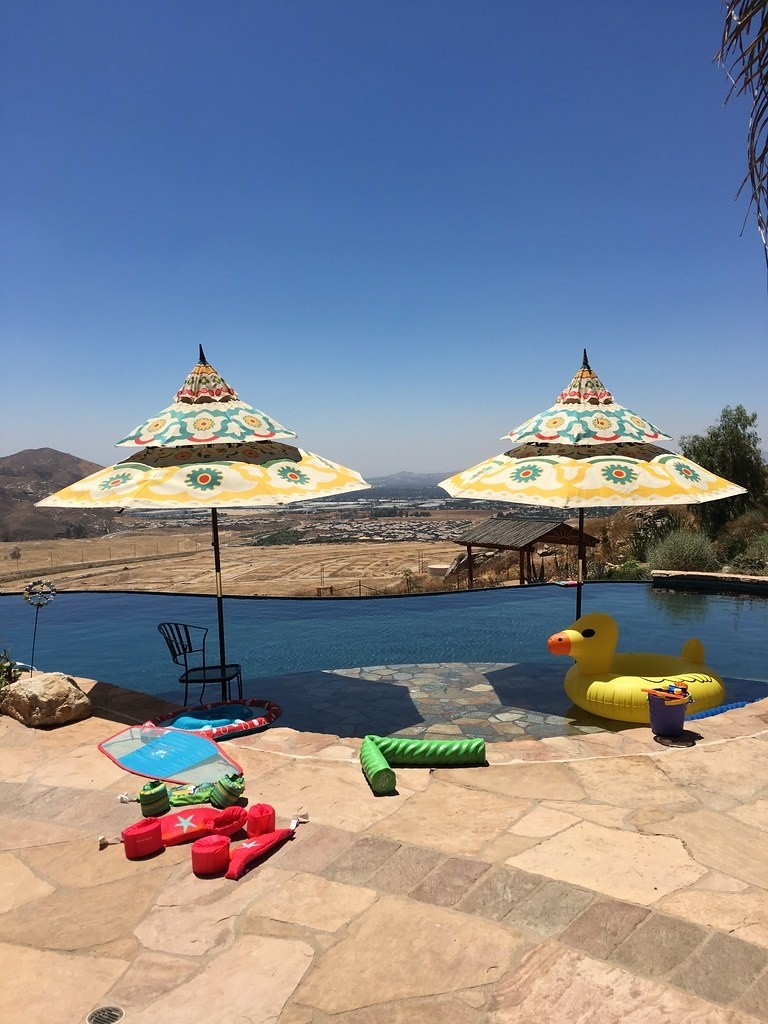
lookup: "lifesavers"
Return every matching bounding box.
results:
[359,734,485,792]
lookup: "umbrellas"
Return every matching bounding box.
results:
[438,348,748,621]
[35,344,373,702]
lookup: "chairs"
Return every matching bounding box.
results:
[157,622,243,707]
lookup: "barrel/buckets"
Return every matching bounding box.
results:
[649,688,692,739]
